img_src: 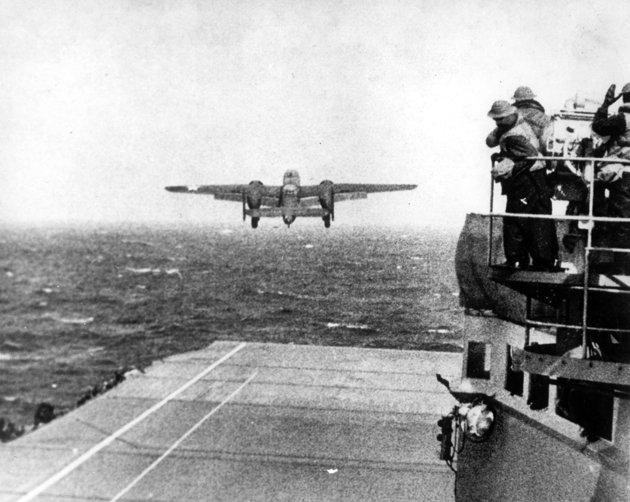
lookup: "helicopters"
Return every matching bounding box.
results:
[166,169,417,230]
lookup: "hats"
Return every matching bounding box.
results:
[508,85,538,101]
[618,83,630,93]
[486,100,519,119]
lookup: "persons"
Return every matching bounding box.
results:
[487,85,552,270]
[590,81,630,274]
[486,100,560,273]
